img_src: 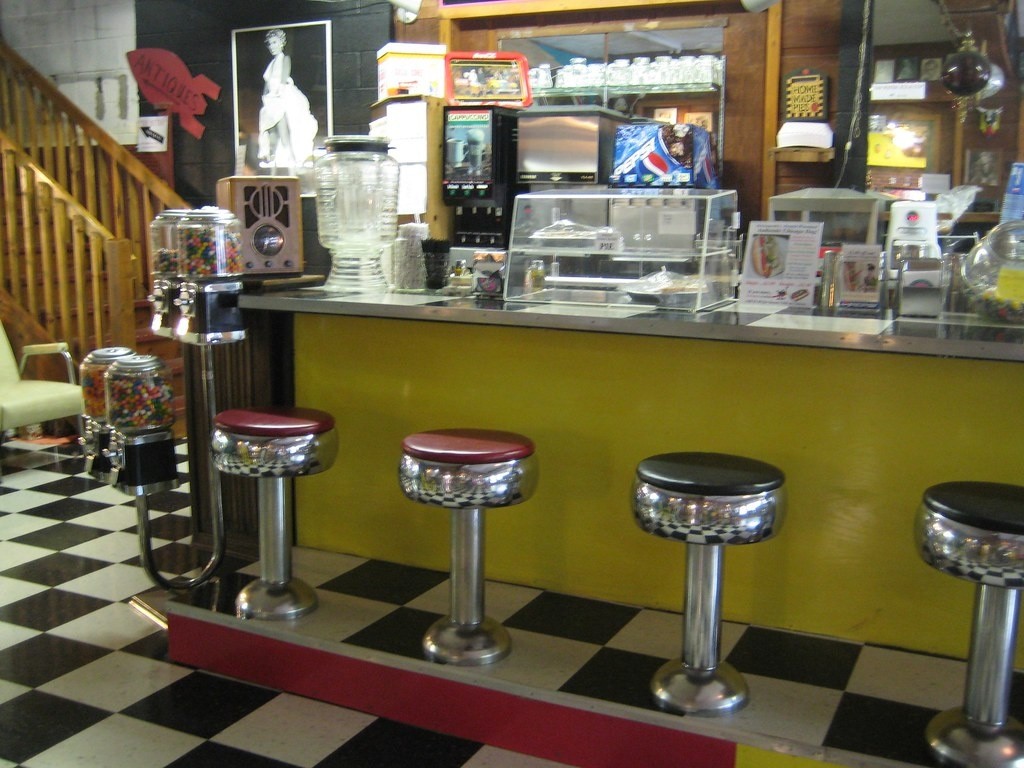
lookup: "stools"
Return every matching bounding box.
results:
[914,482,1024,767]
[631,452,787,718]
[209,407,339,619]
[397,429,536,667]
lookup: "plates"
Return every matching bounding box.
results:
[527,234,597,248]
[622,290,708,306]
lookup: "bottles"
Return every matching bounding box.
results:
[311,133,402,292]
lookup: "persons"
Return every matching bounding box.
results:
[256,28,318,176]
[468,67,504,96]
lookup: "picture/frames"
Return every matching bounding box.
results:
[446,51,533,109]
[864,57,1002,197]
[231,20,335,198]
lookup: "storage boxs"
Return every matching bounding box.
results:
[368,43,453,244]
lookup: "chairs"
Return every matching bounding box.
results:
[0,319,86,449]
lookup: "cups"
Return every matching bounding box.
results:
[467,137,484,168]
[537,63,553,88]
[555,55,720,87]
[420,253,450,289]
[447,138,469,163]
[394,235,426,289]
[613,128,681,187]
[997,162,1024,224]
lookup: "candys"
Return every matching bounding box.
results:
[79,361,175,432]
[150,221,243,278]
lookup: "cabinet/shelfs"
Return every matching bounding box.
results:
[502,188,739,312]
